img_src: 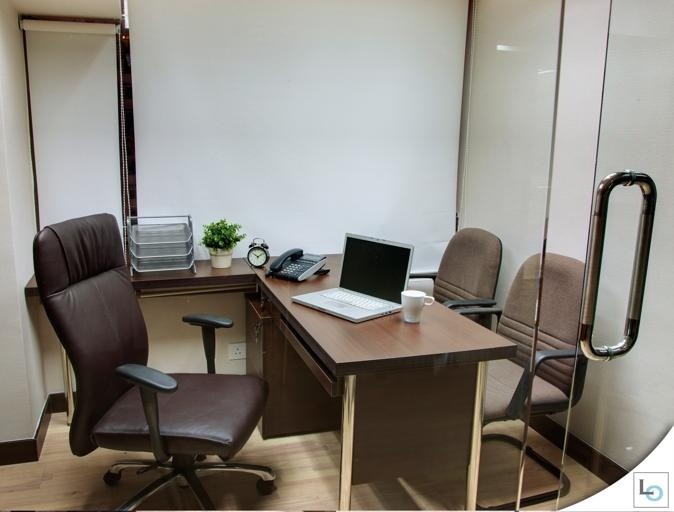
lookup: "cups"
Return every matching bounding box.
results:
[401,289,434,324]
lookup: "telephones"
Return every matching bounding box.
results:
[270,248,327,282]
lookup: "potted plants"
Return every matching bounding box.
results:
[198,217,248,269]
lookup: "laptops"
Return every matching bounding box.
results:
[290,233,414,324]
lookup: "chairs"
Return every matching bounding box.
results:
[409,227,589,511]
[34,213,276,511]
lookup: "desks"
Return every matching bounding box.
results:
[25,250,519,510]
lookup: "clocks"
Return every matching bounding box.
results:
[247,236,271,270]
